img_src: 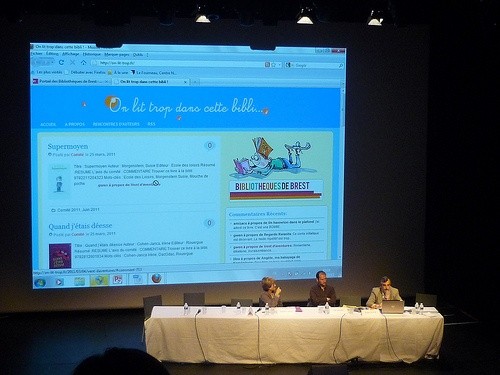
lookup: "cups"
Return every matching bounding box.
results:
[222,306,226,313]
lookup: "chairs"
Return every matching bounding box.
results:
[184,292,205,305]
[414,293,437,307]
[362,297,369,305]
[230,298,254,307]
[141,294,162,343]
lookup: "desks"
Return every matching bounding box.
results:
[144,305,444,365]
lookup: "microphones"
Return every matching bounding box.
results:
[196,309,201,315]
[255,308,261,313]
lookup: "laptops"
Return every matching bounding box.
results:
[379,300,404,314]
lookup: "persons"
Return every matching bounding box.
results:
[366,276,403,308]
[309,271,336,307]
[259,277,282,307]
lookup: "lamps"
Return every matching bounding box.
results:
[237,5,254,27]
[368,9,384,26]
[192,1,212,24]
[296,2,316,24]
[160,4,174,25]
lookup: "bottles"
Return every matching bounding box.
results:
[415,302,419,314]
[325,302,329,314]
[419,303,424,315]
[264,303,269,315]
[236,302,241,314]
[184,303,189,315]
